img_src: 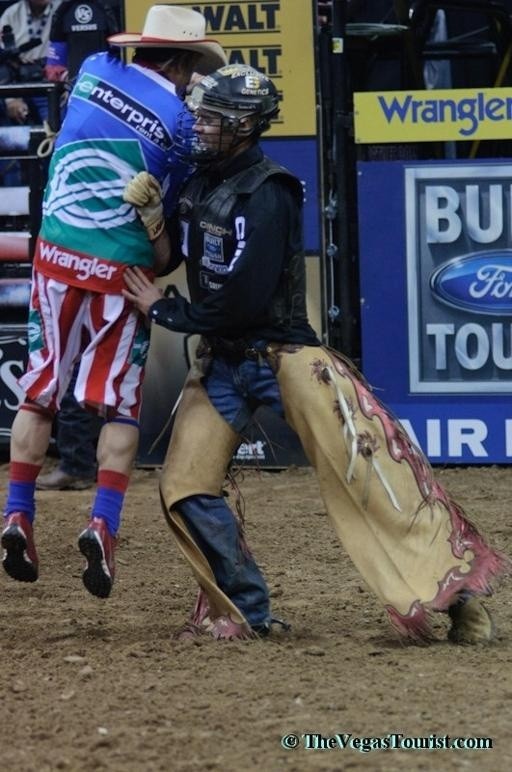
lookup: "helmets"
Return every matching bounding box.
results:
[176,63,280,172]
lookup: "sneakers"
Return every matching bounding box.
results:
[448,594,494,648]
[2,513,38,582]
[77,516,119,599]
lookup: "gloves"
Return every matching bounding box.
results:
[122,169,168,240]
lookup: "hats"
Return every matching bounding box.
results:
[106,4,230,77]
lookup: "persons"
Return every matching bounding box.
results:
[1,2,128,195]
[115,63,502,646]
[33,325,103,494]
[1,4,232,601]
